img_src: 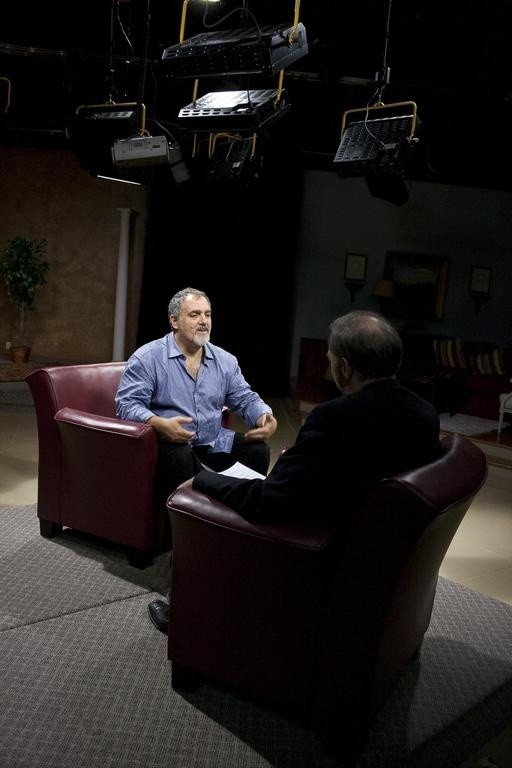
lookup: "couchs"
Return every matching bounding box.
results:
[295,329,510,434]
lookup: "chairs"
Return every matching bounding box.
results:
[495,389,512,433]
[161,427,491,724]
[22,355,238,572]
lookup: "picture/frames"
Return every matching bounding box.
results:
[467,265,493,294]
[343,251,371,282]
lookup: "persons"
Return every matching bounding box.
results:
[114,286,279,487]
[145,308,441,637]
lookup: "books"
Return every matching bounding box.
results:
[432,339,503,375]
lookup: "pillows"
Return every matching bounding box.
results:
[465,347,506,378]
[429,337,470,372]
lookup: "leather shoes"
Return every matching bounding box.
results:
[146,599,171,635]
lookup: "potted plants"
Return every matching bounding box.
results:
[0,231,55,367]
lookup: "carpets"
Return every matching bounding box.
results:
[435,410,511,437]
[1,492,178,633]
[2,573,511,766]
